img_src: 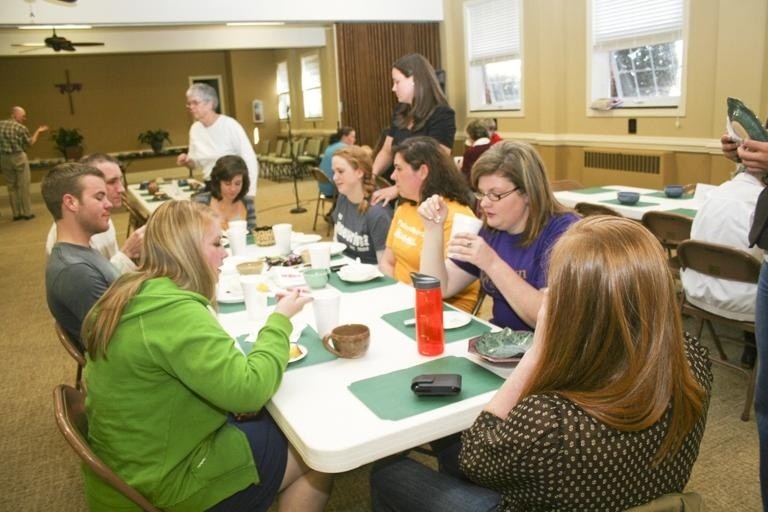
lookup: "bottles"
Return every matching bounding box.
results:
[409,270,445,357]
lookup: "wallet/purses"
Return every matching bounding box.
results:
[410,373,462,397]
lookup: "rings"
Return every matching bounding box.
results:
[467,240,472,248]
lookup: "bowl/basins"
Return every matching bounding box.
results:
[617,192,640,205]
[236,262,265,275]
[665,185,684,197]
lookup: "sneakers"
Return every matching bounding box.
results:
[740,344,758,371]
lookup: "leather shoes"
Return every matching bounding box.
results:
[13,214,35,221]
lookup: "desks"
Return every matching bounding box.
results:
[124,177,535,478]
[28,145,194,189]
[549,182,701,235]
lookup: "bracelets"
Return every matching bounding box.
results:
[371,173,377,177]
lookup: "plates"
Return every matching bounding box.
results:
[288,346,307,364]
[291,231,380,282]
[466,329,534,364]
[443,311,472,329]
[725,95,766,144]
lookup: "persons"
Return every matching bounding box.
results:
[369,214,714,512]
[460,120,494,192]
[678,163,768,370]
[721,114,768,511]
[369,53,456,207]
[42,162,121,357]
[0,106,48,221]
[318,127,357,225]
[379,136,494,322]
[176,83,259,197]
[195,155,256,236]
[483,118,503,146]
[80,200,334,512]
[45,152,147,276]
[331,145,394,265]
[416,140,584,334]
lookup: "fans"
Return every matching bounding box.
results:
[11,28,110,61]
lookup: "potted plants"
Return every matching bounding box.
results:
[51,126,88,160]
[137,128,175,152]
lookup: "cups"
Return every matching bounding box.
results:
[302,268,330,288]
[241,275,272,323]
[141,176,200,201]
[227,219,247,256]
[323,322,371,358]
[273,223,292,255]
[313,296,339,339]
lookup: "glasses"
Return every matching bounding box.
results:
[185,100,204,108]
[470,185,520,202]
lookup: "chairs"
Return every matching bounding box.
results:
[50,323,88,390]
[676,240,762,423]
[46,378,165,512]
[637,210,731,364]
[572,201,624,220]
[257,132,329,184]
[627,491,716,511]
[306,164,342,239]
[373,175,397,207]
